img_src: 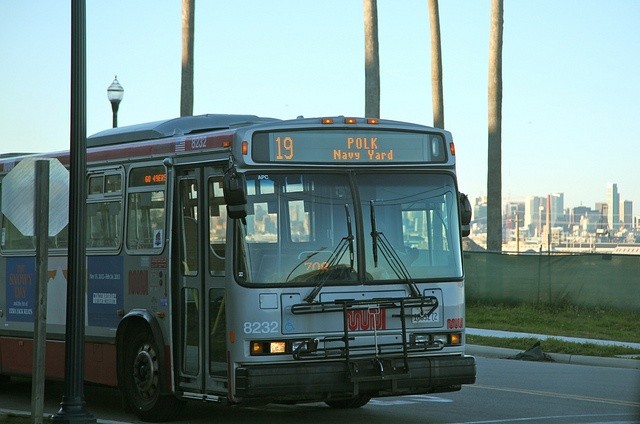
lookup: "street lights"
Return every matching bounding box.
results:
[107,76,124,128]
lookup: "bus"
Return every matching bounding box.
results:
[1,114,475,408]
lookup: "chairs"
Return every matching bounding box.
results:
[89,237,118,247]
